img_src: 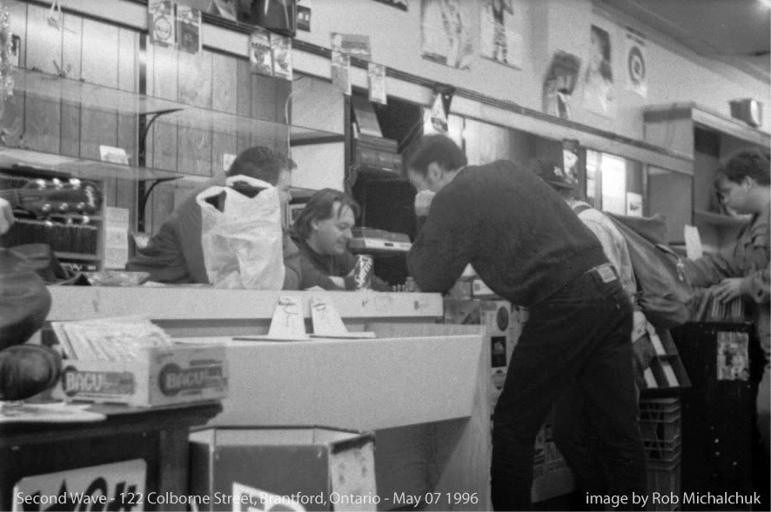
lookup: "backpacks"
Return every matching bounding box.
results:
[573,204,696,328]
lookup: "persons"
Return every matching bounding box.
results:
[123,132,770,512]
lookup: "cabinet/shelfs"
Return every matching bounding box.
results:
[0,61,347,235]
[644,105,770,246]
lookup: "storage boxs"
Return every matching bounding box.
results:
[50,343,231,411]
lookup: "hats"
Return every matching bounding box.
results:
[522,157,575,189]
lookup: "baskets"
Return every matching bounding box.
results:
[637,396,683,508]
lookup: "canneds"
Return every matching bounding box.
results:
[354,253,374,291]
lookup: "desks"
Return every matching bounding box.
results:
[0,399,224,512]
